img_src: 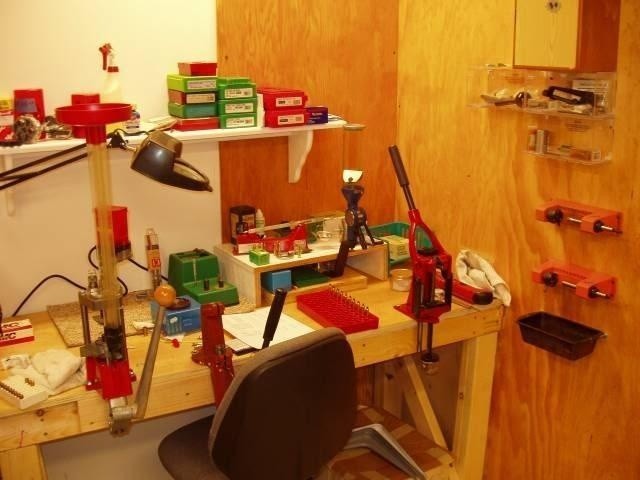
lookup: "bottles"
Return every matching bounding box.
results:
[13,89,46,140]
[255,208,265,228]
[100,65,125,132]
[71,94,100,138]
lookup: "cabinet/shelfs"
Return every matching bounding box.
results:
[513,0,620,73]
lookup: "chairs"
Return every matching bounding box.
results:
[158,326,359,480]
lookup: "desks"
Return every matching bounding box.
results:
[0,259,505,480]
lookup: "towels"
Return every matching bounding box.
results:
[455,249,511,307]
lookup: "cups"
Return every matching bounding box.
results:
[294,239,306,257]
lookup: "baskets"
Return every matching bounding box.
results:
[367,222,432,265]
